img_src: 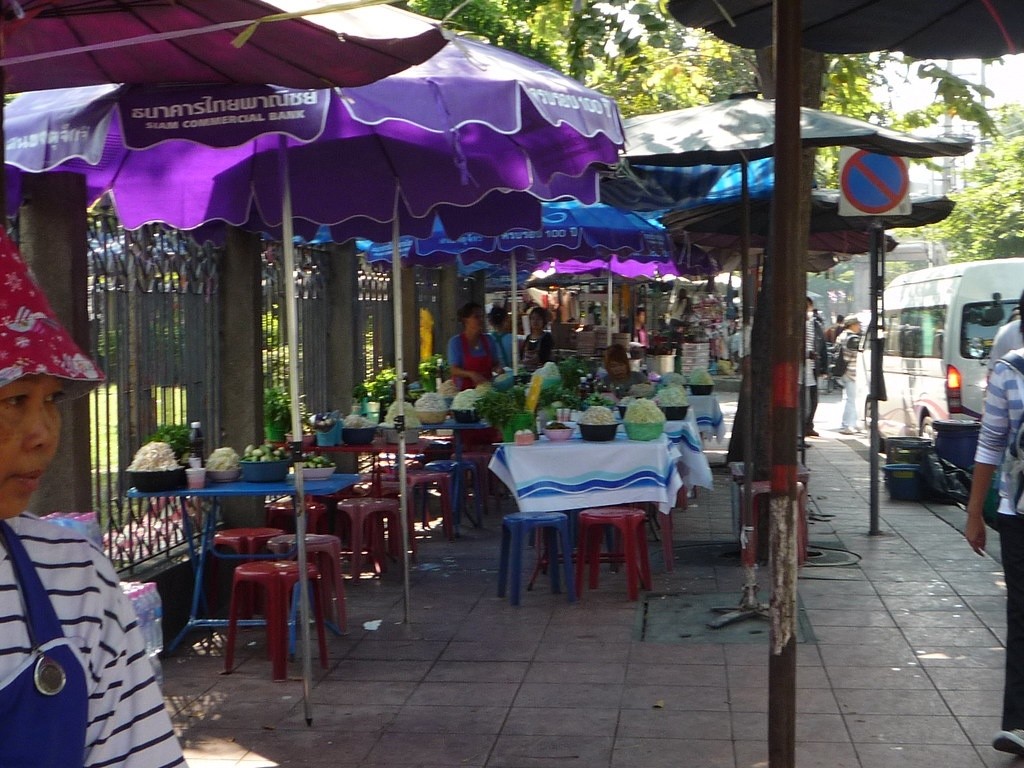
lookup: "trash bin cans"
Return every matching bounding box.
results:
[932,419,981,473]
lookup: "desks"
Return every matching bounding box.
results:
[124,387,726,661]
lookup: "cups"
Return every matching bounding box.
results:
[185,468,206,489]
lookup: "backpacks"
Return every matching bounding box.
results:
[827,333,855,377]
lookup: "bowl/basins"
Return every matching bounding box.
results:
[207,470,242,482]
[302,467,337,480]
[238,461,293,482]
[616,405,691,420]
[284,434,314,445]
[543,429,573,440]
[341,427,377,444]
[515,434,533,445]
[578,423,621,441]
[380,422,422,443]
[689,385,714,395]
[125,466,186,493]
[415,409,482,423]
[622,421,664,441]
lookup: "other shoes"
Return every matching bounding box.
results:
[807,430,819,436]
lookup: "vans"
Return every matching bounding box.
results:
[842,257,1024,452]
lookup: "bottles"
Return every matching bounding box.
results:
[555,409,584,421]
[188,422,205,467]
[36,513,162,697]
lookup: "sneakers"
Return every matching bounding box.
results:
[992,728,1024,755]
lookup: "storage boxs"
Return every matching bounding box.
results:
[683,342,710,351]
[681,357,710,363]
[680,363,708,370]
[681,350,710,357]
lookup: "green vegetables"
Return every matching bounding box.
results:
[472,355,615,428]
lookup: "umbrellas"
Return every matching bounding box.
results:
[0,24,630,732]
[0,0,456,97]
[288,83,975,623]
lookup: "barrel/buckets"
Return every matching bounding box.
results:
[887,437,931,464]
[933,419,980,478]
[883,464,920,500]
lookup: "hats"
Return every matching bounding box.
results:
[0,228,105,401]
[843,315,862,326]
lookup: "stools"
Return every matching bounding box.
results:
[267,534,350,634]
[452,451,501,517]
[220,560,332,682]
[332,497,405,585]
[577,509,653,601]
[265,502,328,576]
[405,469,455,541]
[378,452,426,508]
[423,459,483,535]
[496,510,577,604]
[211,528,285,631]
[373,460,422,515]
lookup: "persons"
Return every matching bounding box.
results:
[804,296,829,436]
[830,315,845,347]
[835,314,862,435]
[965,287,1024,755]
[595,344,647,399]
[0,227,190,767]
[447,302,505,391]
[519,307,556,372]
[668,288,691,327]
[487,305,512,368]
[634,307,650,350]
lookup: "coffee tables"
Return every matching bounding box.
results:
[355,481,418,563]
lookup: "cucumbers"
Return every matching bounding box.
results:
[241,444,287,461]
[302,455,336,466]
